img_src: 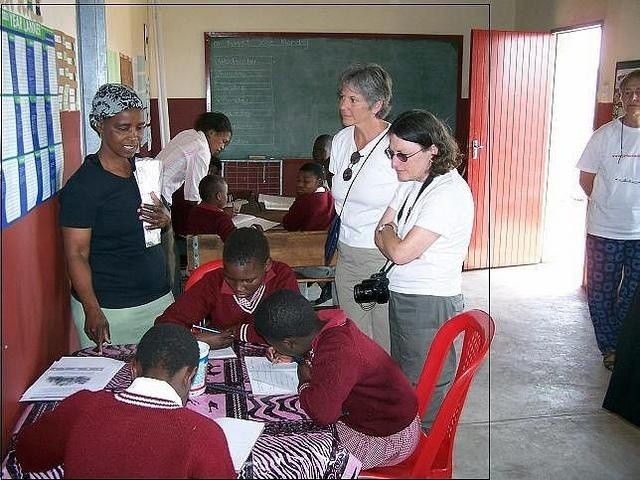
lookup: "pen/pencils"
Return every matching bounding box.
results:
[272,355,282,366]
[193,325,236,338]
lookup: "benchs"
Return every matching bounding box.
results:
[181,231,341,308]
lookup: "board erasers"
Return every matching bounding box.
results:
[249,156,267,159]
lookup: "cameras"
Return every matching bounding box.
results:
[354,272,390,304]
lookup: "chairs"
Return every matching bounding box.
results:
[184,260,225,291]
[358,309,496,480]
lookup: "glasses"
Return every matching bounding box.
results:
[342,149,365,182]
[384,144,426,163]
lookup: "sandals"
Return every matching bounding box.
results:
[601,350,614,371]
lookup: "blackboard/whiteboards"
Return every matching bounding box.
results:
[204,33,464,161]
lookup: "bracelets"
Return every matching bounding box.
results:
[378,224,394,234]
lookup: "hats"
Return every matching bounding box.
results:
[88,82,146,128]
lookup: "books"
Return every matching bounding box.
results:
[19,346,299,475]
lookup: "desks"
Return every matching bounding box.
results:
[226,196,290,231]
[3,342,362,480]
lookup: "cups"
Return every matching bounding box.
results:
[183,340,211,400]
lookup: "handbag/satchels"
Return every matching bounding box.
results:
[323,213,341,267]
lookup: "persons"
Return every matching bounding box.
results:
[56,84,176,350]
[329,62,402,358]
[575,70,640,370]
[154,225,300,349]
[374,109,476,434]
[153,113,338,245]
[16,323,238,480]
[251,289,422,470]
